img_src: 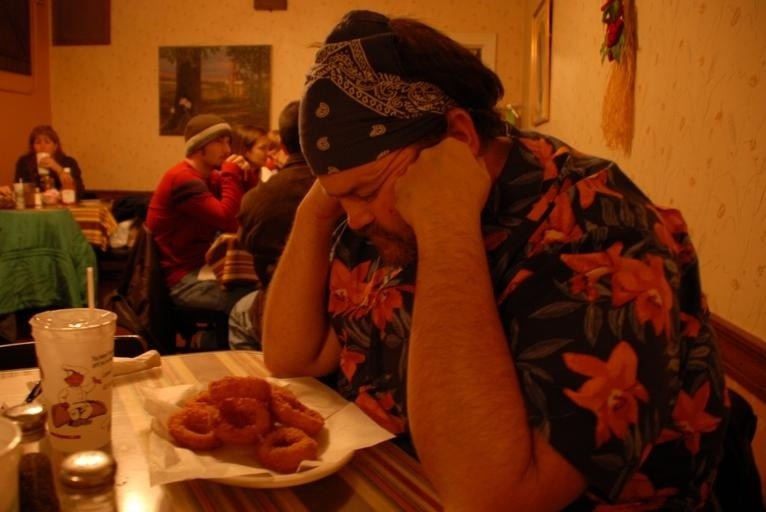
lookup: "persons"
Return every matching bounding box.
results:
[14,126,84,200]
[262,10,729,512]
[228,100,316,351]
[146,115,289,348]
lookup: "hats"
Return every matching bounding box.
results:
[183,112,234,159]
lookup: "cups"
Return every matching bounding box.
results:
[42,190,59,204]
[36,153,50,175]
[29,308,120,455]
[14,183,26,209]
[236,159,249,172]
[0,412,23,511]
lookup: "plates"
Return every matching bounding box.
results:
[207,377,358,487]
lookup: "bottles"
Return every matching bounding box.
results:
[4,403,59,512]
[33,187,41,208]
[60,448,112,512]
[62,167,76,205]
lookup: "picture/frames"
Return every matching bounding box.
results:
[447,32,497,77]
[530,0,553,129]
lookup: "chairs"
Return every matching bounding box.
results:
[0,188,258,366]
[703,303,764,512]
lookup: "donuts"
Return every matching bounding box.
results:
[170,376,325,474]
